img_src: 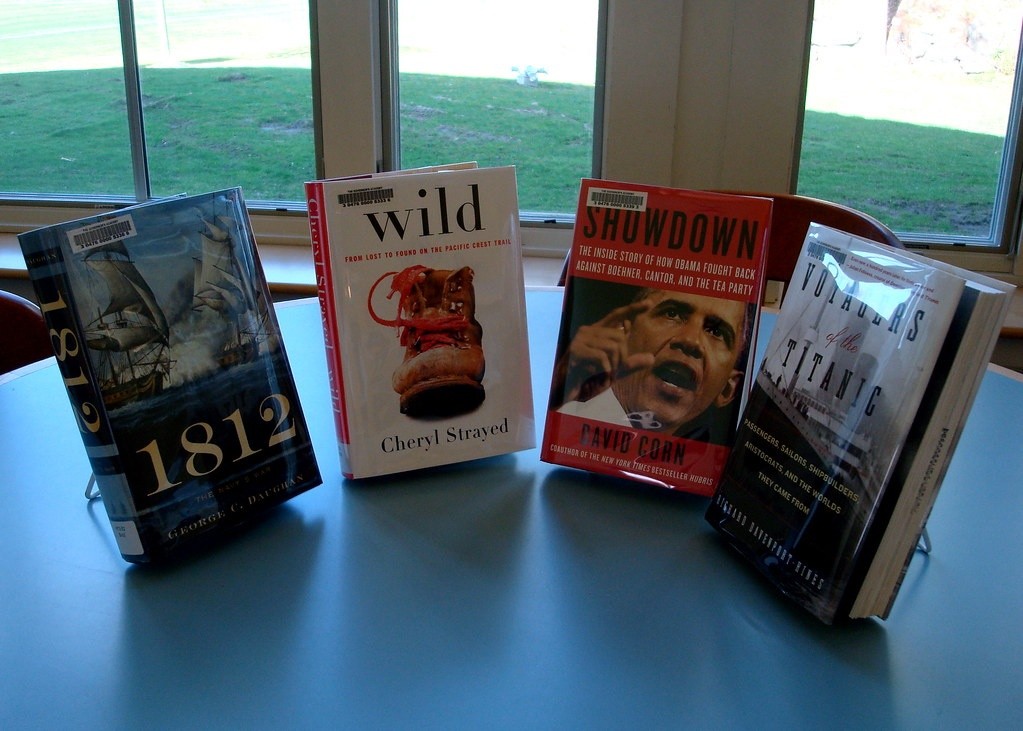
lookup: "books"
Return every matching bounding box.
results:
[17,186,323,564]
[539,178,774,498]
[703,222,1018,631]
[303,161,536,479]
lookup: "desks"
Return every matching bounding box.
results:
[0,281,1022,730]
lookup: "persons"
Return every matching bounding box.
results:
[548,286,749,436]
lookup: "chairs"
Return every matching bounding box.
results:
[555,191,906,310]
